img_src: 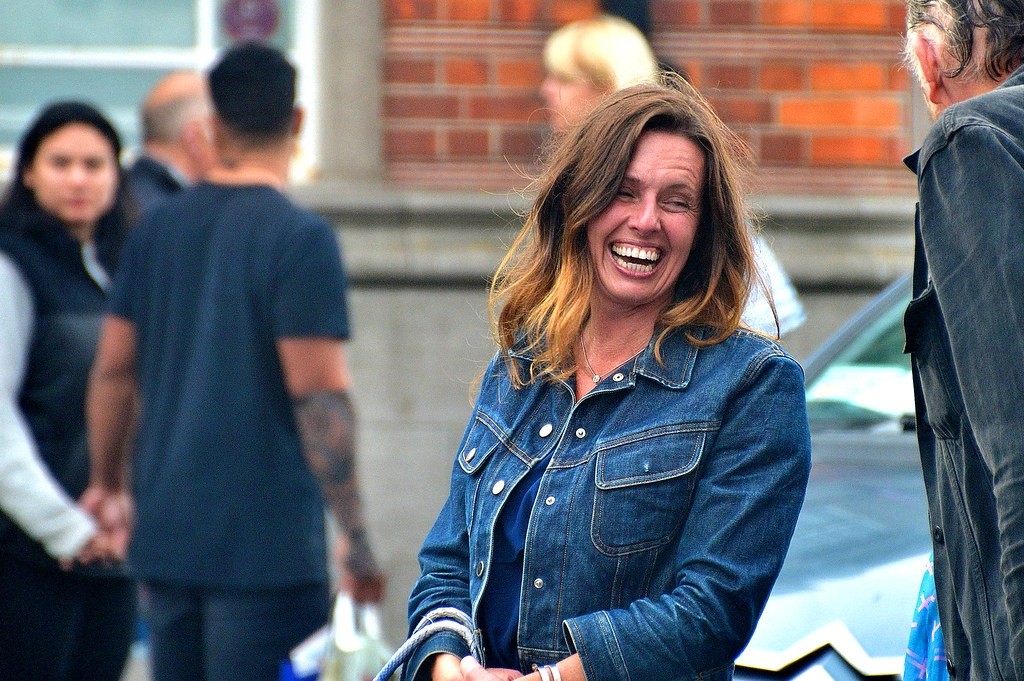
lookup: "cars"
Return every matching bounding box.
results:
[729,272,931,681]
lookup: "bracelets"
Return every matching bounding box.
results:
[534,661,563,681]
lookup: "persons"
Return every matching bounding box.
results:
[896,0,1024,681]
[74,38,383,678]
[102,74,220,267]
[0,99,143,681]
[533,15,659,133]
[406,81,810,681]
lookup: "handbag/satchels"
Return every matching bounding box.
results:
[736,235,809,340]
[283,589,402,681]
